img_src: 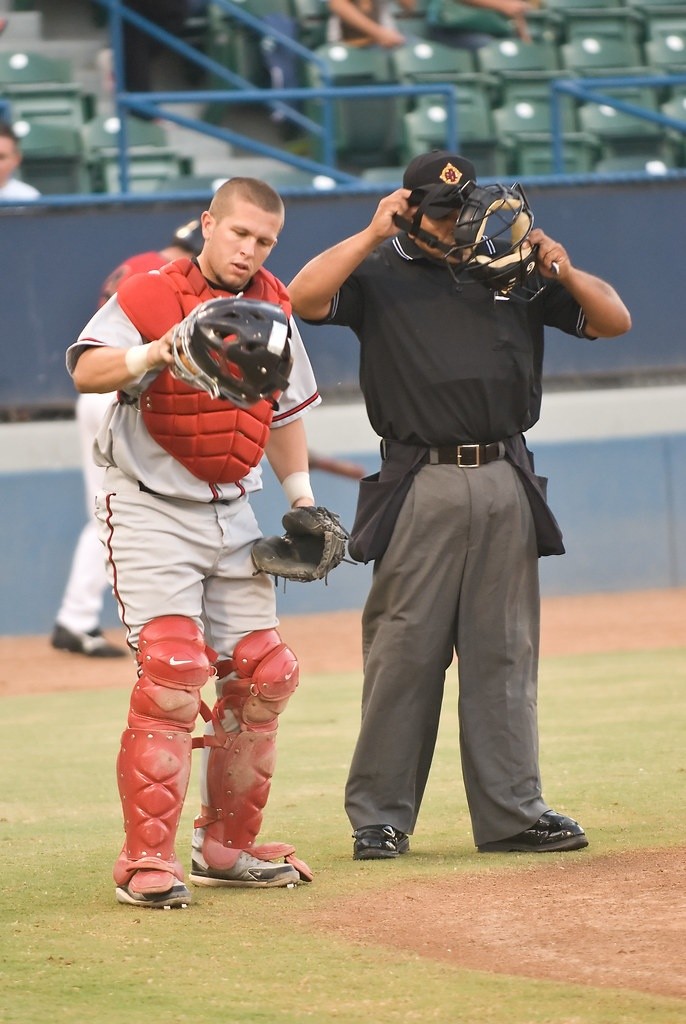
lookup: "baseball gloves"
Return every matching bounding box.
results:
[251,505,348,581]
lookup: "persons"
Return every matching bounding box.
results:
[65,176,350,909]
[53,218,209,658]
[92,0,188,121]
[0,124,41,202]
[285,151,633,860]
[320,0,545,51]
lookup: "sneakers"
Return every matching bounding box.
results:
[116,875,191,910]
[188,839,300,888]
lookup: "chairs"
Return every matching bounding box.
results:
[0,45,179,194]
[213,0,686,168]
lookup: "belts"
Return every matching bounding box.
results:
[381,439,508,468]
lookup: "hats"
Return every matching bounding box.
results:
[403,149,476,219]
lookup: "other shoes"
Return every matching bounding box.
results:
[52,625,129,658]
[477,810,589,852]
[351,824,410,859]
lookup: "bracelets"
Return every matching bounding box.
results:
[124,340,156,377]
[282,471,315,506]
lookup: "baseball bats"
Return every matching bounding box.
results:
[305,450,365,480]
